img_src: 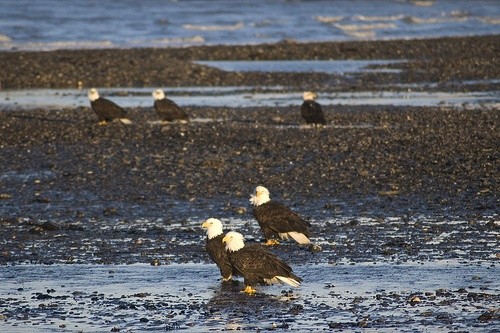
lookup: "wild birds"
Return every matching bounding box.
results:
[201,218,304,297]
[301,91,326,130]
[87,88,128,125]
[250,187,313,246]
[152,87,190,125]
